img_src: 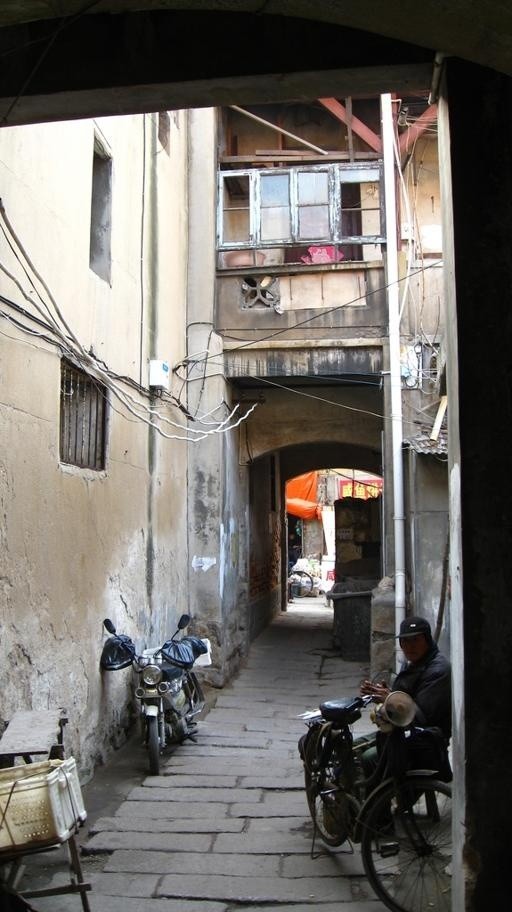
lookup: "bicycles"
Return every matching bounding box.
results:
[288,562,313,598]
[298,692,452,912]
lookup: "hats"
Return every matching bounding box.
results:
[393,616,431,639]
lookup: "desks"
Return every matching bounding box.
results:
[0,707,69,768]
[0,839,92,912]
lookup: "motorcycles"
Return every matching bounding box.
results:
[104,614,206,775]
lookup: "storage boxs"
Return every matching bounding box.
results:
[142,638,211,667]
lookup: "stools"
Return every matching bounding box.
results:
[404,778,442,822]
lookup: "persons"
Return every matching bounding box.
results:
[330,615,455,837]
